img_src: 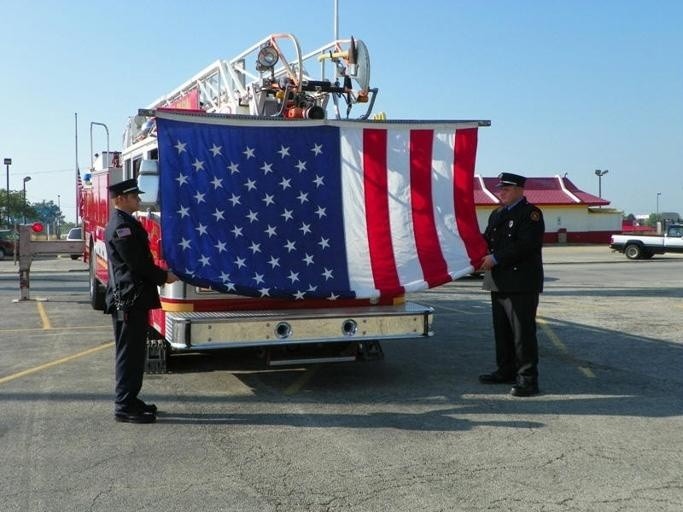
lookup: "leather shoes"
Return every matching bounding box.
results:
[117,404,157,422]
[511,386,539,396]
[480,373,516,383]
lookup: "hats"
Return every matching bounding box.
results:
[496,174,527,187]
[107,179,145,196]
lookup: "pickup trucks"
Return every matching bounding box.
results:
[609,222,683,259]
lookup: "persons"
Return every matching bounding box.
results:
[674,230,681,237]
[478,170,546,398]
[102,176,181,424]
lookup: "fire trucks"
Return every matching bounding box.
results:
[72,0,441,373]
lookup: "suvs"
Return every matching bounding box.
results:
[0,229,22,259]
[66,225,83,259]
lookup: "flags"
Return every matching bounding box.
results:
[152,109,496,304]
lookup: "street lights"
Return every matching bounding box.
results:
[3,157,13,228]
[22,176,32,224]
[594,168,609,208]
[656,191,662,223]
